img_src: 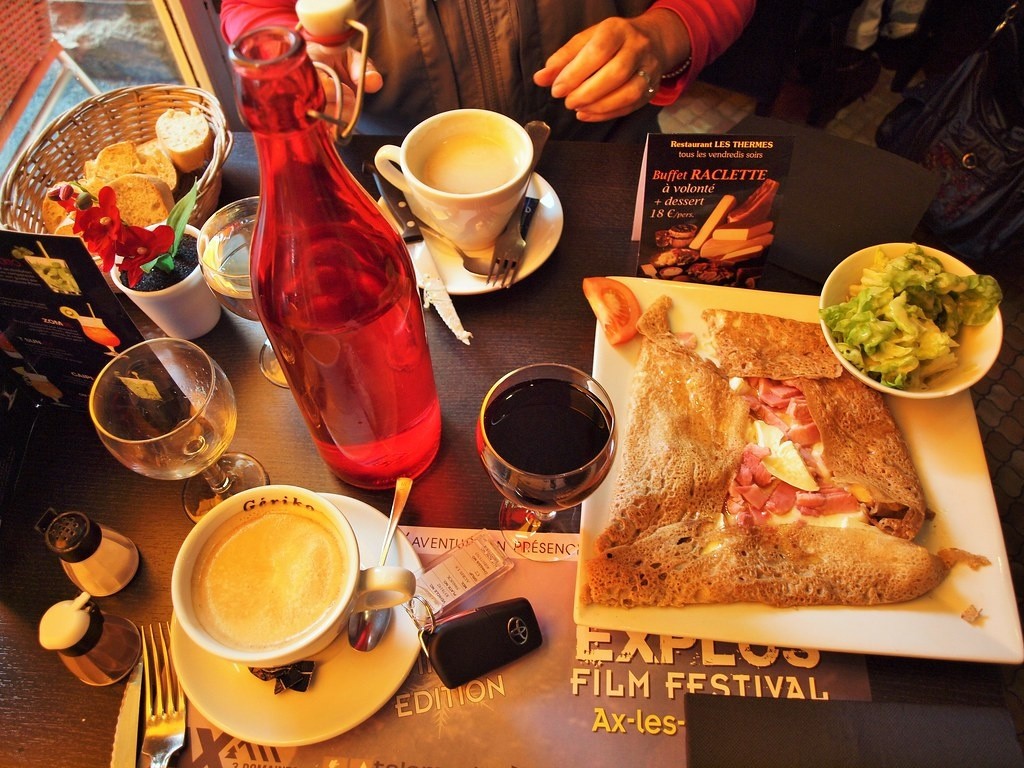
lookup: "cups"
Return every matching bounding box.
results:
[171,485,416,668]
[0,333,24,359]
[374,108,534,251]
[24,256,82,296]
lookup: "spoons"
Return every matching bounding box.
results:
[347,478,413,652]
[412,213,505,277]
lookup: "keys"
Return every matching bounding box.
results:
[418,598,543,689]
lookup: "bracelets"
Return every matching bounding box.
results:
[661,56,692,79]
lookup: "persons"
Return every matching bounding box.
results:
[219,0,756,139]
[754,0,1024,271]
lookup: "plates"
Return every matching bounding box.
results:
[573,277,1024,665]
[171,492,423,748]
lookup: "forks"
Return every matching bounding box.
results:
[485,120,551,289]
[141,621,186,768]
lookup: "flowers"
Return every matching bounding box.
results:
[44,172,200,294]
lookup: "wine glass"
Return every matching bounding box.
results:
[0,383,17,410]
[27,373,70,407]
[89,336,271,524]
[77,316,128,358]
[475,363,617,562]
[197,196,290,390]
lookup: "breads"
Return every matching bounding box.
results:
[41,106,214,235]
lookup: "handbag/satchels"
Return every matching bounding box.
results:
[875,47,1024,265]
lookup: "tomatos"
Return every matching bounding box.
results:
[582,277,642,346]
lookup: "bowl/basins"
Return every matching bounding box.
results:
[819,243,1003,399]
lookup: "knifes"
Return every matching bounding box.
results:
[109,654,143,768]
[361,155,471,345]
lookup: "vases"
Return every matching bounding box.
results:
[109,219,222,345]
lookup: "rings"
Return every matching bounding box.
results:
[637,71,656,94]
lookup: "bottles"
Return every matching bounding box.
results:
[38,592,140,686]
[45,511,138,596]
[228,0,442,490]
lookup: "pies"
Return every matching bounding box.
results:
[580,297,990,609]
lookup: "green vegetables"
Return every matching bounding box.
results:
[819,244,1003,389]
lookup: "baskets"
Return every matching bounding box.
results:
[0,83,235,293]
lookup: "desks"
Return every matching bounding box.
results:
[1,130,1024,768]
